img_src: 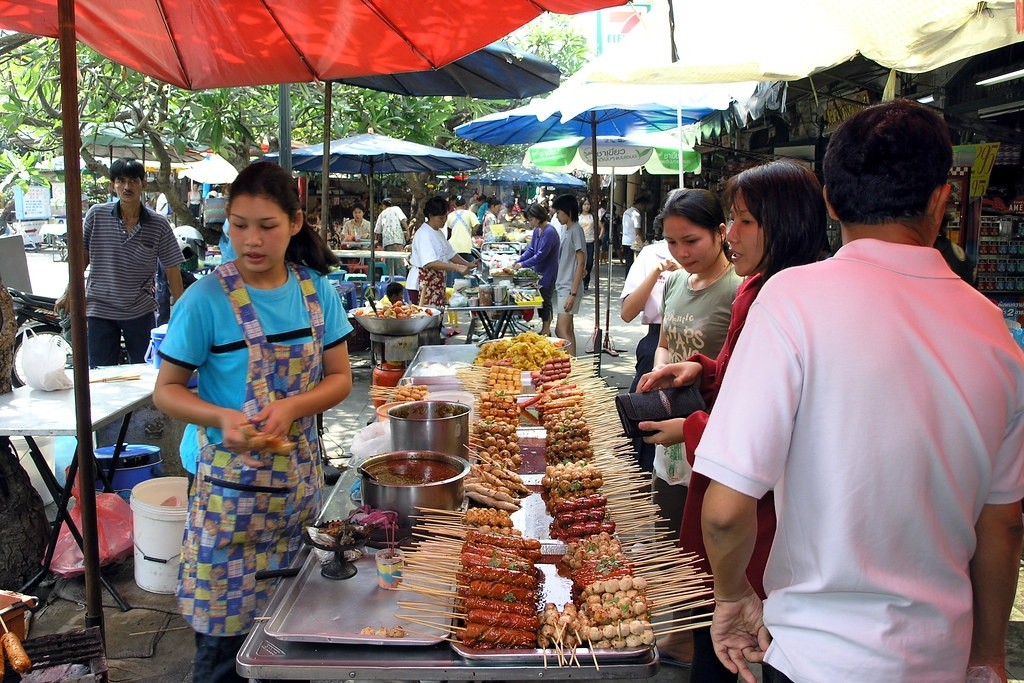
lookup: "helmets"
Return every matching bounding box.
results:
[173,225,204,258]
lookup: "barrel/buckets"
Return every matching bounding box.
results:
[493,275,513,286]
[373,363,405,407]
[490,285,508,306]
[144,329,167,369]
[93,443,164,504]
[129,477,189,594]
[9,437,78,506]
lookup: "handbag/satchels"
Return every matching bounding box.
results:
[653,442,692,487]
[41,467,135,577]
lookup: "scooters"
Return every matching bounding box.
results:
[6,240,212,388]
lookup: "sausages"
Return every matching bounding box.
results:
[454,532,542,651]
[549,493,616,541]
[520,357,584,421]
[571,559,634,591]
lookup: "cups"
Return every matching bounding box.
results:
[375,548,405,589]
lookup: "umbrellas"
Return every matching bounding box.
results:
[263,127,484,300]
[79,119,309,207]
[454,104,716,377]
[0,0,631,683]
[315,46,560,274]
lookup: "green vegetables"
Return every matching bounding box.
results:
[513,268,537,277]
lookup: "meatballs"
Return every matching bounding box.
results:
[540,407,603,507]
[462,506,522,536]
[537,577,654,650]
[474,419,522,470]
[561,533,623,566]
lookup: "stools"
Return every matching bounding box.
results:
[328,262,411,309]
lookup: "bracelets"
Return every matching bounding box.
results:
[569,292,577,296]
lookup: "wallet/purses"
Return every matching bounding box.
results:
[616,384,708,436]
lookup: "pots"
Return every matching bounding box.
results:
[358,450,471,527]
[386,400,472,461]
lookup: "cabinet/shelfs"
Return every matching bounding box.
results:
[978,214,1024,292]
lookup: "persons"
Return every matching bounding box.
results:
[597,200,622,266]
[187,184,201,223]
[619,160,974,683]
[511,187,604,357]
[155,191,168,218]
[1,190,16,235]
[107,192,119,202]
[206,185,218,198]
[146,198,151,205]
[691,97,1024,683]
[339,192,517,335]
[622,196,648,280]
[152,162,357,683]
[222,185,228,198]
[54,158,187,369]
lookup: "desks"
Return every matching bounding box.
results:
[449,304,543,339]
[333,250,410,282]
[1,363,199,611]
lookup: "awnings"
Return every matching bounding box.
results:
[588,1,1024,85]
[535,64,789,147]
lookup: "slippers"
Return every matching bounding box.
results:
[655,642,692,668]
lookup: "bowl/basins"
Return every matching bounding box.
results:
[424,391,475,418]
[464,289,479,294]
[376,401,407,423]
[468,299,479,307]
[349,305,442,335]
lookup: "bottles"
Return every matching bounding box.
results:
[479,284,491,306]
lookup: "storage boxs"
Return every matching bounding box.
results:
[0,589,39,640]
